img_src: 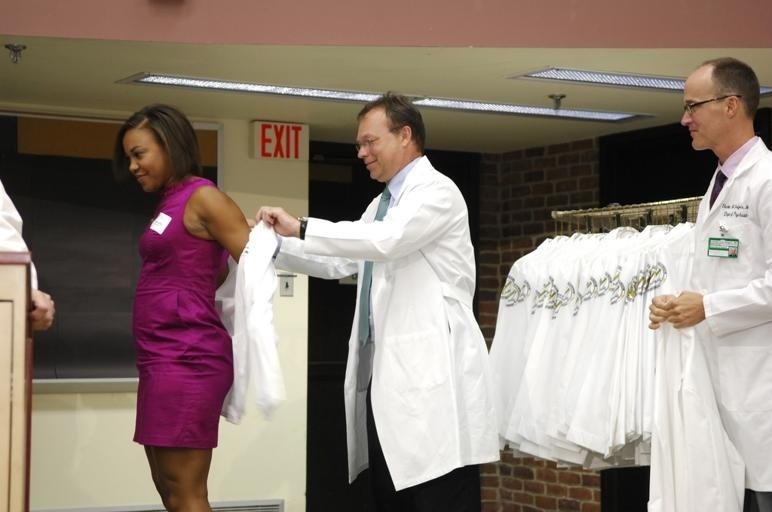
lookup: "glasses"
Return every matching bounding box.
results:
[354,135,385,149]
[685,94,743,112]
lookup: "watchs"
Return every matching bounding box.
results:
[298,213,307,238]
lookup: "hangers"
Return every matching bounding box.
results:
[554,196,702,242]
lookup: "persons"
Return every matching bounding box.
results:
[116,102,283,512]
[647,58,772,510]
[239,91,504,512]
[0,185,56,333]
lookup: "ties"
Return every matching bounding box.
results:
[708,170,728,210]
[354,185,391,344]
